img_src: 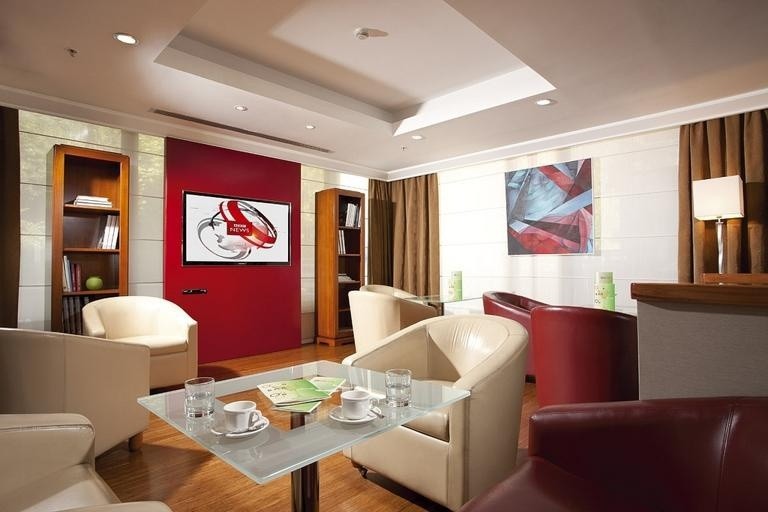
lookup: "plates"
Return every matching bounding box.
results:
[217,428,271,449]
[210,416,269,438]
[328,405,383,425]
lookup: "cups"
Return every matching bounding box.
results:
[223,399,263,431]
[182,375,216,419]
[185,416,216,445]
[385,368,415,409]
[340,390,379,419]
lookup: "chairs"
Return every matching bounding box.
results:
[0,326,152,458]
[359,283,430,306]
[81,295,199,389]
[528,305,638,407]
[340,312,530,511]
[456,396,767,511]
[348,290,438,352]
[0,413,175,512]
[484,290,552,376]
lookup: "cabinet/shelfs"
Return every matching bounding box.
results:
[314,186,366,347]
[50,143,131,334]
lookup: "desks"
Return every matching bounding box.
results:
[136,358,471,511]
[404,294,483,315]
[630,281,767,402]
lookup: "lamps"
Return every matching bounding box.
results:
[691,175,745,285]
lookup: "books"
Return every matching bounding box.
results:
[257,379,332,406]
[273,375,347,414]
[61,194,119,336]
[338,204,362,282]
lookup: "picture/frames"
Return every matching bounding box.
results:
[181,189,293,268]
